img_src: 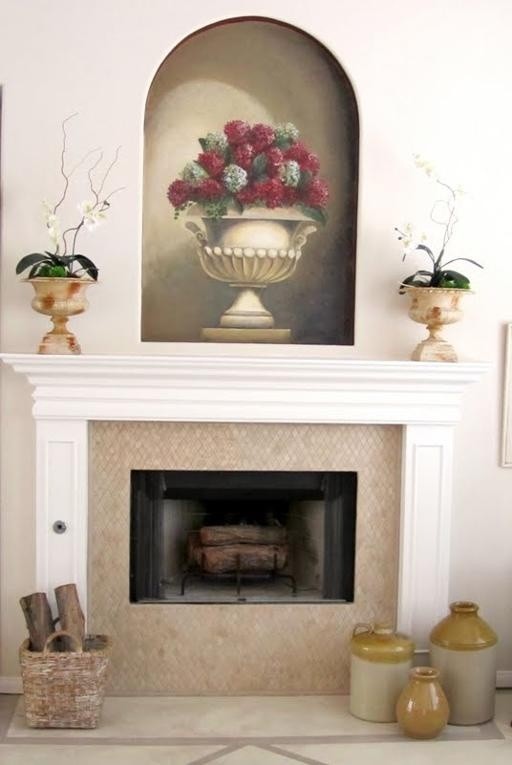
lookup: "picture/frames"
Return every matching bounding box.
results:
[500,323,512,468]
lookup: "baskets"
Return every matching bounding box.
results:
[19,633,112,729]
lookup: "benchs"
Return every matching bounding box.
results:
[182,522,297,595]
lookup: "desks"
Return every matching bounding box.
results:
[186,528,200,571]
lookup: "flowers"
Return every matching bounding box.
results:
[394,153,483,295]
[16,113,124,281]
[167,119,330,226]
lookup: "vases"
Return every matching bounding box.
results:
[25,277,96,355]
[396,665,451,741]
[407,289,463,362]
[185,215,317,328]
